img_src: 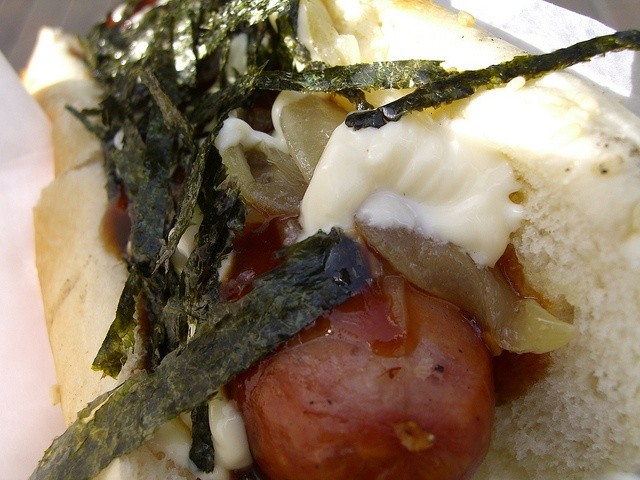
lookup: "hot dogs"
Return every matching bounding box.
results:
[17,0,638,478]
